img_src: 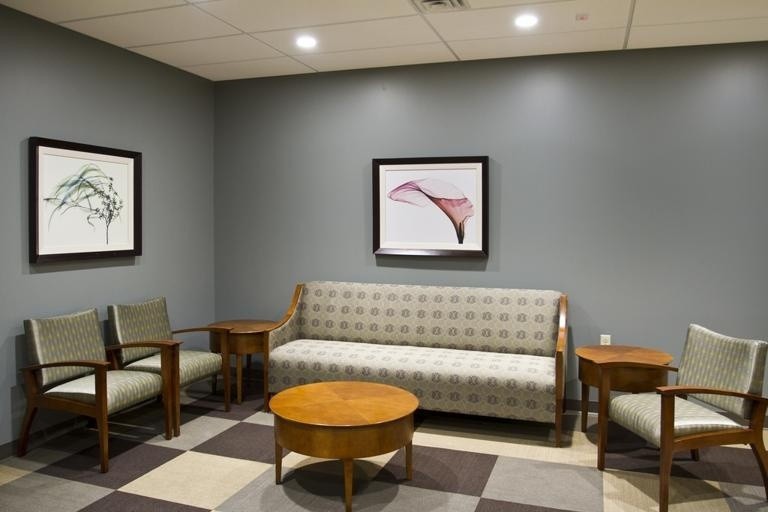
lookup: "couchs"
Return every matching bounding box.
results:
[262,279,568,448]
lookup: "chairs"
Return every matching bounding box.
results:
[16,305,177,473]
[102,295,234,436]
[595,324,768,512]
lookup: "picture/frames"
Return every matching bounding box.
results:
[373,154,490,258]
[27,137,143,262]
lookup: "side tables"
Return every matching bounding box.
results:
[573,346,674,433]
[208,320,276,404]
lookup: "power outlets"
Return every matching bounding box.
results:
[601,334,611,346]
